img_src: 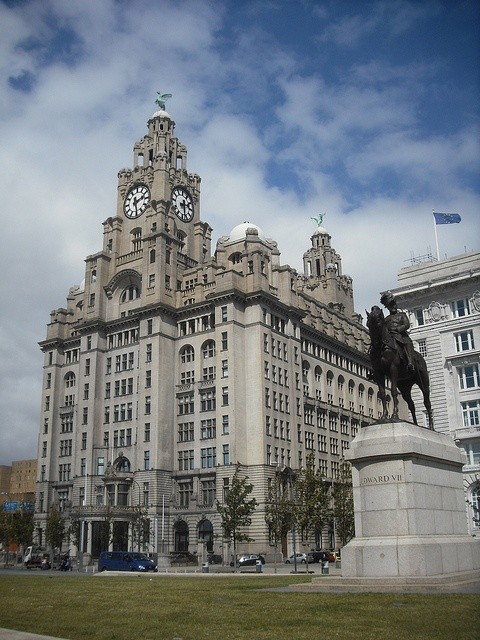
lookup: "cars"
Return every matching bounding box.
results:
[326,553,336,563]
[236,553,265,565]
[309,551,329,563]
[284,552,313,563]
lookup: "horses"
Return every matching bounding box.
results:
[364,308,435,431]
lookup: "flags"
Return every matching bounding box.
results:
[432,212,461,227]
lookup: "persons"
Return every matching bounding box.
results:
[381,290,416,376]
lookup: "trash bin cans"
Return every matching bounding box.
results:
[256,559,262,573]
[321,560,329,574]
[202,561,209,573]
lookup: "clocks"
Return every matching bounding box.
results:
[171,185,195,222]
[123,185,151,219]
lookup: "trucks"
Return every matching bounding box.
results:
[24,545,46,569]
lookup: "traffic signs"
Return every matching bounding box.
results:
[2,501,22,513]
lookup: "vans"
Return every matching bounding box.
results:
[98,551,157,572]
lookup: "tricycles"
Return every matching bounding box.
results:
[39,553,52,570]
[57,554,73,571]
